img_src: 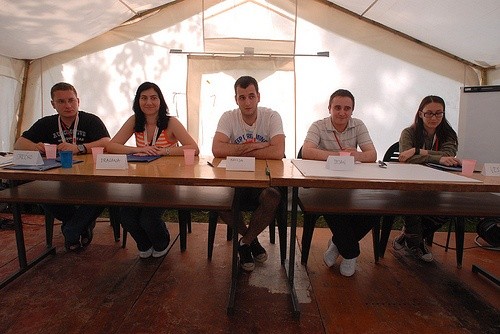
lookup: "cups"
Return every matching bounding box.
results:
[339,151,351,156]
[91,147,104,162]
[462,160,476,176]
[44,145,57,158]
[59,151,73,168]
[183,149,196,165]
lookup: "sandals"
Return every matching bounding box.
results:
[61,228,81,252]
[81,220,95,248]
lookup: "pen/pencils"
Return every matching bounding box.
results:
[207,161,215,167]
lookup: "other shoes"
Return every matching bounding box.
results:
[152,234,171,257]
[140,247,151,258]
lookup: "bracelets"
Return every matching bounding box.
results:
[83,145,88,154]
[165,148,169,156]
[415,147,420,154]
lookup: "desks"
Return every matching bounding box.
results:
[266,159,500,320]
[0,153,271,316]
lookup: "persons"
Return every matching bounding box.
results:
[212,75,285,271]
[14,82,111,252]
[301,89,377,276]
[103,82,199,259]
[392,96,462,264]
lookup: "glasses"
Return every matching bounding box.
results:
[421,111,444,118]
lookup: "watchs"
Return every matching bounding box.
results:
[77,144,85,155]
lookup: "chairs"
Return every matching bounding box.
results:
[44,141,464,267]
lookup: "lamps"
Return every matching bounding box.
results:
[474,218,500,250]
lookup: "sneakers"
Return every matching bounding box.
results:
[393,230,406,250]
[339,258,355,276]
[415,240,433,261]
[249,239,268,262]
[323,239,339,268]
[236,238,255,271]
